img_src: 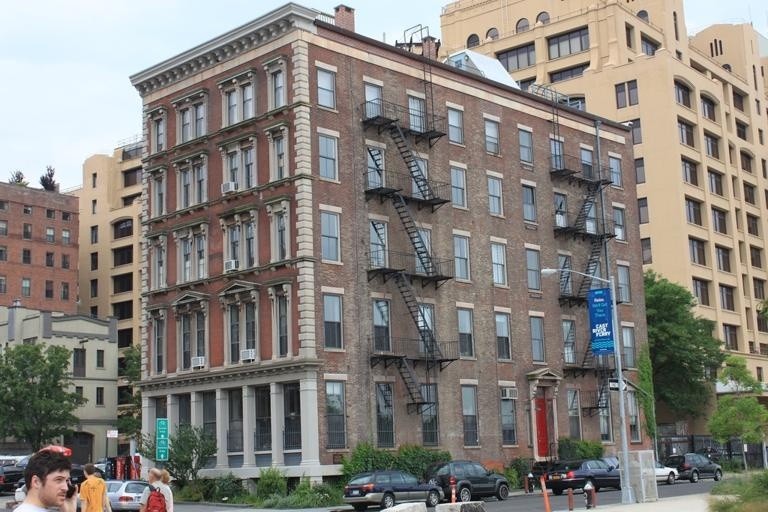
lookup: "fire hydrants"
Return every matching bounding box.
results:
[590,484,596,507]
[522,474,529,493]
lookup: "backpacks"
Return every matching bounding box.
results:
[146,483,166,512]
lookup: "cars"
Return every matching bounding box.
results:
[344,461,510,510]
[105,480,151,512]
[0,455,30,493]
[550,453,722,494]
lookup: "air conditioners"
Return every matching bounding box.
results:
[224,260,239,271]
[191,357,205,368]
[221,182,237,195]
[240,348,255,360]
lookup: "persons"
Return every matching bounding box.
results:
[138,467,170,512]
[93,467,112,512]
[161,468,174,512]
[10,449,79,512]
[78,463,107,512]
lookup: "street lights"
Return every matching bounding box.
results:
[539,269,631,505]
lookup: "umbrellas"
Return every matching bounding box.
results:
[30,443,73,459]
